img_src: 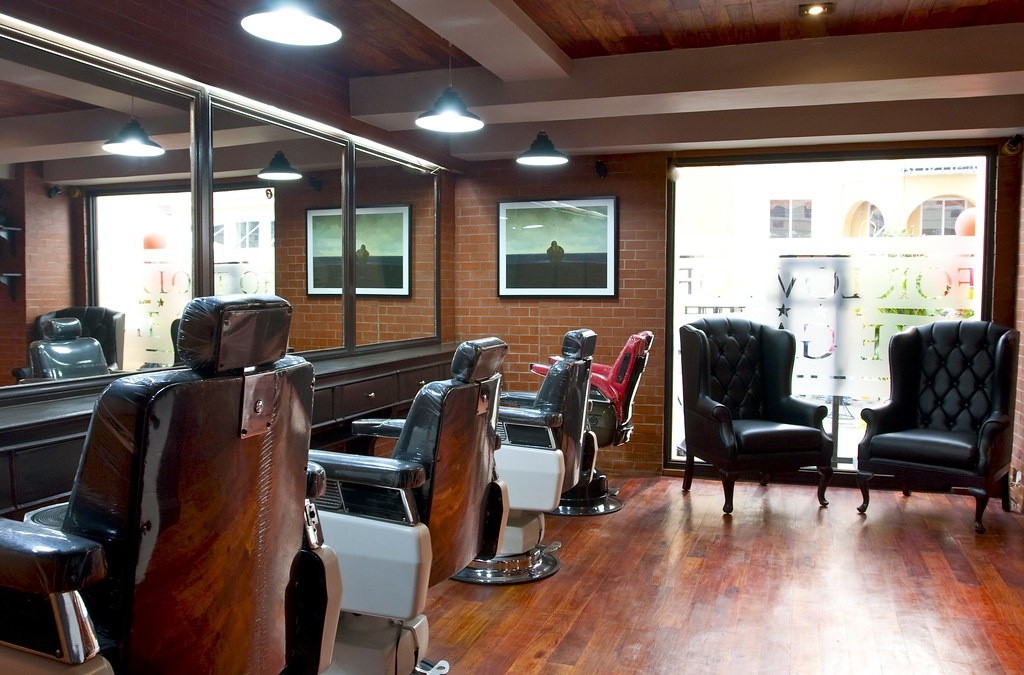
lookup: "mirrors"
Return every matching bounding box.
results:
[204,93,350,360]
[0,24,204,408]
[350,141,441,358]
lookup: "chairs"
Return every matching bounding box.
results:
[18,317,124,385]
[679,316,834,516]
[308,336,510,675]
[0,294,344,675]
[11,304,126,383]
[529,331,654,517]
[856,319,1021,532]
[448,327,599,587]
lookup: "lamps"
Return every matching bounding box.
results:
[415,43,485,132]
[515,127,569,167]
[239,0,343,48]
[101,97,166,157]
[257,148,303,181]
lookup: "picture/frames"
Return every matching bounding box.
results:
[304,202,412,299]
[496,194,619,300]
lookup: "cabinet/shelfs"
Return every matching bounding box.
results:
[0,213,22,303]
